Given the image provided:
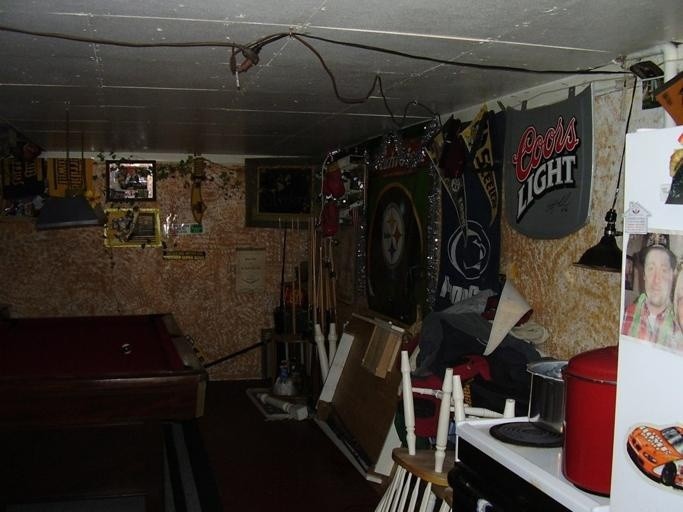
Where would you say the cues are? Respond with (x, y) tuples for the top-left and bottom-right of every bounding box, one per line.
(275, 217), (338, 409)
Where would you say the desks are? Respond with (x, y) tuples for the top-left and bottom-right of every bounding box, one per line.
(1, 312), (209, 511)
(447, 415), (610, 512)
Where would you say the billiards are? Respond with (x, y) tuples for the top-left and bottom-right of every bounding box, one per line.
(120, 343), (131, 354)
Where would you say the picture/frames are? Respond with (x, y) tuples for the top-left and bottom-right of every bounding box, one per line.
(353, 162), (443, 329)
(103, 159), (163, 247)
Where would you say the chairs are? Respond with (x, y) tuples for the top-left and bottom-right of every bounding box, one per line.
(372, 349), (517, 512)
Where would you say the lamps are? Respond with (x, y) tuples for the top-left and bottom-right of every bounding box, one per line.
(36, 99), (101, 233)
(572, 73), (640, 272)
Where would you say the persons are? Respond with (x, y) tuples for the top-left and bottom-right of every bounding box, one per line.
(672, 269), (683, 332)
(620, 243), (682, 346)
